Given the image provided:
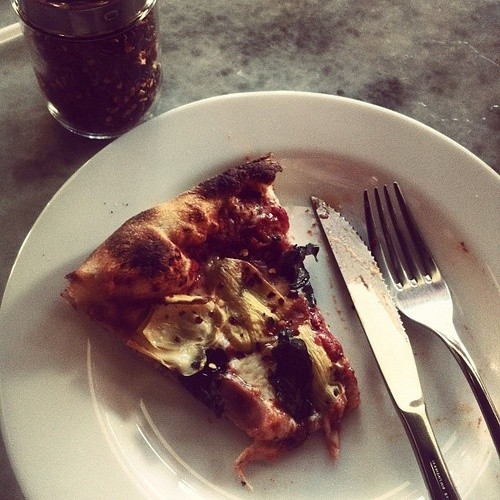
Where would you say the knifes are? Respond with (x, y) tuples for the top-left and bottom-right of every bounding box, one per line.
(308, 193), (465, 500)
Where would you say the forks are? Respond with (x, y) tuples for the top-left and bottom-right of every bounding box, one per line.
(362, 182), (499, 458)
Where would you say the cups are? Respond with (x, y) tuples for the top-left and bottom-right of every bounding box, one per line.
(8, 0), (166, 143)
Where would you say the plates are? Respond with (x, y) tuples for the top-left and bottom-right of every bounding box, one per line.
(1, 86), (500, 499)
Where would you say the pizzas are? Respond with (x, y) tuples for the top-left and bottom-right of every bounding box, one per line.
(60, 153), (361, 494)
